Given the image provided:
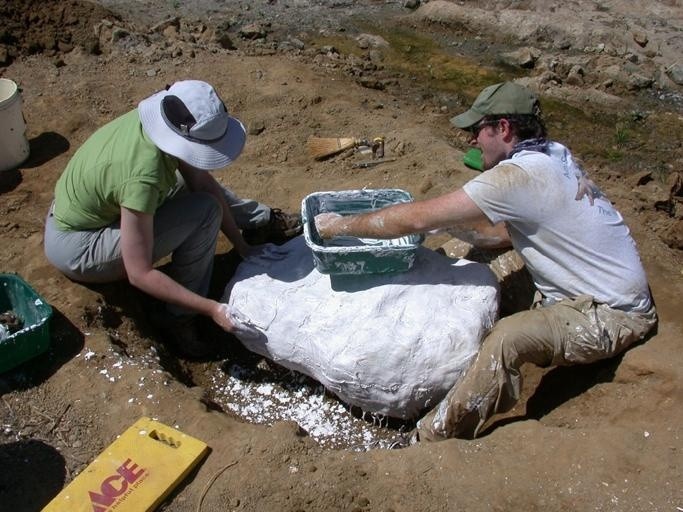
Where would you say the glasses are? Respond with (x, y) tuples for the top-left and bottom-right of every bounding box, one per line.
(469, 119), (500, 138)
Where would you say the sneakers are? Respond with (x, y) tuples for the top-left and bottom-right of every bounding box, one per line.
(163, 315), (211, 364)
(350, 431), (409, 452)
(241, 208), (302, 245)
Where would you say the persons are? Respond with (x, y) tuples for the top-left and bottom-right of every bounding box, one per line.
(43, 78), (303, 359)
(311, 80), (658, 454)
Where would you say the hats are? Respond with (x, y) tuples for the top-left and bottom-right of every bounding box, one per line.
(137, 79), (246, 171)
(448, 79), (540, 132)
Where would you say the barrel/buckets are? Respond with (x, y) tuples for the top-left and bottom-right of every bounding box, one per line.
(0, 77), (31, 173)
